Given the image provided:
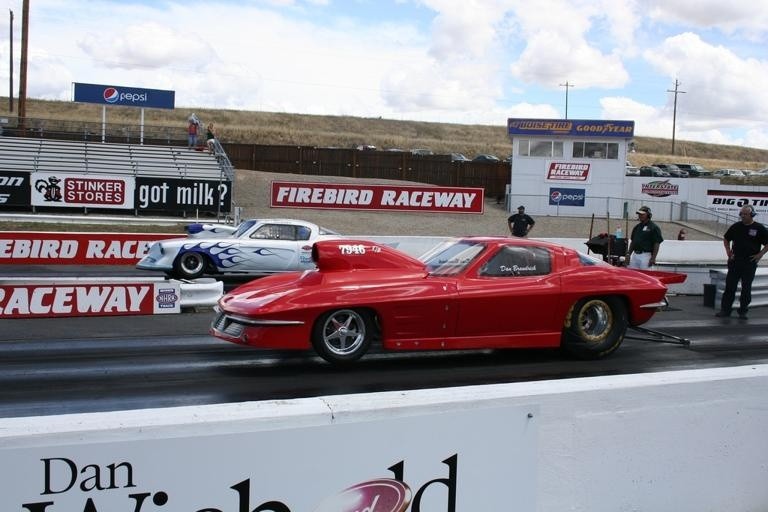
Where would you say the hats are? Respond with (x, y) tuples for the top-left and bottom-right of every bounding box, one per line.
(518, 205), (524, 209)
(635, 205), (651, 215)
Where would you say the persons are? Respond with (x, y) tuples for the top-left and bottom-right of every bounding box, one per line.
(624, 206), (663, 271)
(506, 205), (535, 239)
(715, 204), (768, 320)
(186, 113), (217, 152)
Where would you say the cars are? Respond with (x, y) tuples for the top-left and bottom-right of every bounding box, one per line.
(357, 144), (513, 162)
(626, 161), (768, 178)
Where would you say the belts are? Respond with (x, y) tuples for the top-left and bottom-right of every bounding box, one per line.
(635, 249), (646, 254)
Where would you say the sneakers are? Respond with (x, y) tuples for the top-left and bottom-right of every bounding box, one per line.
(739, 313), (749, 320)
(716, 310), (731, 317)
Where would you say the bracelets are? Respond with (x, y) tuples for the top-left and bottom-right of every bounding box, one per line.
(625, 253), (630, 256)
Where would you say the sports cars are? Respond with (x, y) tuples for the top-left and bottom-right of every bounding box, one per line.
(134, 216), (343, 280)
(208, 237), (691, 364)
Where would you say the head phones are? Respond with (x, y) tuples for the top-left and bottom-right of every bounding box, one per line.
(739, 205), (756, 217)
(647, 208), (652, 219)
